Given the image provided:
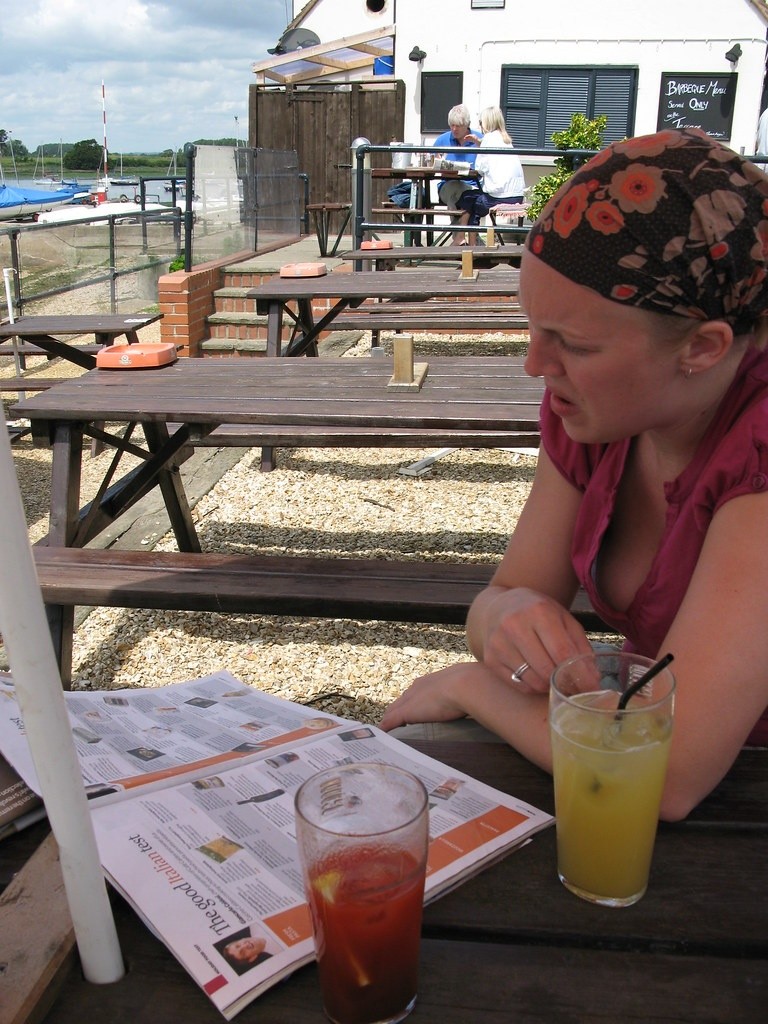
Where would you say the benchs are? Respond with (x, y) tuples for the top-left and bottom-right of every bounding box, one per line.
(2, 303), (545, 447)
(30, 544), (616, 634)
(382, 201), (447, 207)
(306, 203), (380, 257)
(369, 208), (467, 216)
(488, 203), (532, 246)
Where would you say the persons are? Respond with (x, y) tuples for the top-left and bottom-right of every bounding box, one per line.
(755, 107), (768, 172)
(431, 104), (484, 240)
(224, 924), (279, 970)
(377, 128), (768, 821)
(450, 106), (525, 246)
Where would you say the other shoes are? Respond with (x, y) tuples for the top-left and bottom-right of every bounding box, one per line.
(459, 242), (469, 246)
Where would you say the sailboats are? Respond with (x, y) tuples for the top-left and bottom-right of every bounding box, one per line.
(0, 129), (247, 227)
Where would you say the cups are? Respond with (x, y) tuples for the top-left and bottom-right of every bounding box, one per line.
(550, 651), (677, 908)
(294, 762), (431, 1024)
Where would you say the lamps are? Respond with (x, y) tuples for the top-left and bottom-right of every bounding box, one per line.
(725, 43), (742, 63)
(408, 46), (426, 62)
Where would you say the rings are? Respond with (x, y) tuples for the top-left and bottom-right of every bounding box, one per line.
(511, 663), (529, 682)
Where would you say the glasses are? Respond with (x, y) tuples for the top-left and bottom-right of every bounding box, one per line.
(479, 119), (483, 125)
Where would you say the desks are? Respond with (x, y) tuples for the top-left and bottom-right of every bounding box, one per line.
(245, 270), (522, 475)
(8, 354), (546, 692)
(0, 737), (768, 1024)
(0, 312), (165, 457)
(373, 168), (506, 246)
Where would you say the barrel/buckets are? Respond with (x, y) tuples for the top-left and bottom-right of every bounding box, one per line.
(390, 142), (413, 169)
(374, 56), (394, 75)
